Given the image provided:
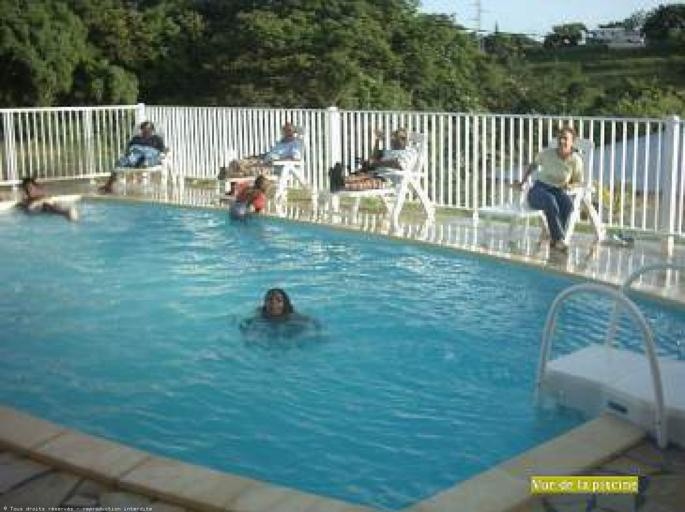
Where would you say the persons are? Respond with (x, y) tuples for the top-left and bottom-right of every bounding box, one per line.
(13, 178), (82, 222)
(217, 120), (303, 182)
(228, 175), (272, 222)
(240, 285), (321, 346)
(327, 129), (418, 197)
(510, 125), (585, 253)
(96, 119), (167, 195)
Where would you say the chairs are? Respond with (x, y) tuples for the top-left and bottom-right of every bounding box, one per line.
(473, 136), (607, 253)
(216, 132), (435, 228)
(113, 124), (176, 198)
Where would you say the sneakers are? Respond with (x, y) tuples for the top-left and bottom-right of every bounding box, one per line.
(326, 162), (346, 193)
(549, 238), (570, 253)
